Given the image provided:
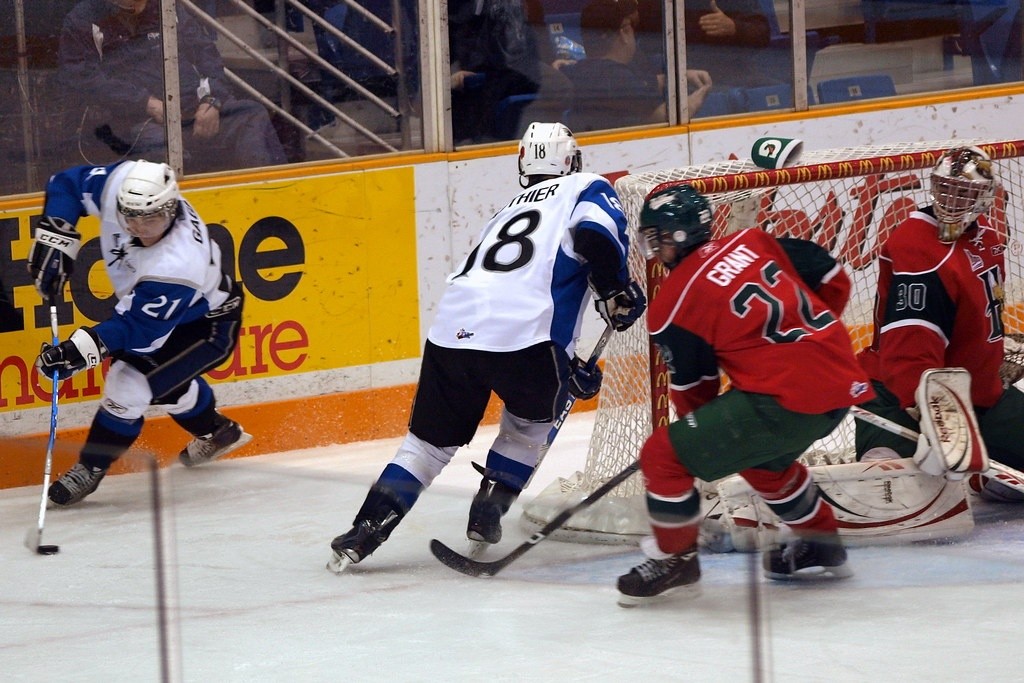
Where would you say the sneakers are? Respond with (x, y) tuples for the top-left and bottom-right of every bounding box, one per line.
(178, 414), (253, 467)
(762, 532), (854, 580)
(325, 484), (405, 576)
(615, 537), (702, 608)
(44, 454), (120, 509)
(965, 473), (1024, 504)
(466, 461), (523, 560)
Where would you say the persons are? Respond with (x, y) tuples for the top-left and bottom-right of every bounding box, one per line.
(29, 160), (251, 504)
(312, 0), (774, 140)
(331, 122), (646, 563)
(852, 145), (1024, 504)
(57, 0), (291, 175)
(618, 185), (878, 598)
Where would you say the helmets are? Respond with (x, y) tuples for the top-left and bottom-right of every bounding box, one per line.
(639, 183), (713, 270)
(116, 159), (180, 237)
(928, 144), (995, 245)
(517, 121), (582, 189)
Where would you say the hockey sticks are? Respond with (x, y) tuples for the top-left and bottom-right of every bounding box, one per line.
(425, 461), (641, 580)
(32, 282), (63, 555)
(849, 402), (1024, 495)
(469, 306), (620, 491)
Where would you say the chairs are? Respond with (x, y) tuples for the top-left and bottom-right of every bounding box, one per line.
(252, 0), (1024, 144)
(35, 67), (61, 164)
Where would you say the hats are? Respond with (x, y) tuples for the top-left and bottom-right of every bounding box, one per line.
(578, 0), (638, 43)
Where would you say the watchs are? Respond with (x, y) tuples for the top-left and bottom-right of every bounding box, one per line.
(199, 95), (223, 110)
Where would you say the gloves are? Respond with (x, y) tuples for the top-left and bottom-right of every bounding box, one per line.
(36, 325), (111, 382)
(27, 215), (82, 301)
(567, 350), (603, 401)
(592, 278), (646, 332)
